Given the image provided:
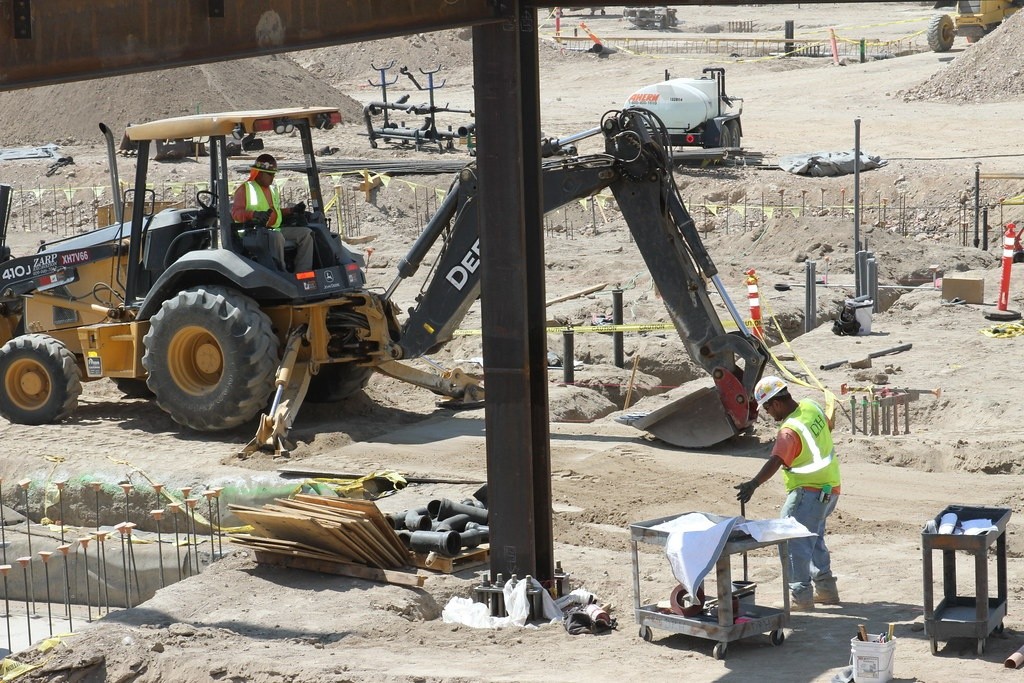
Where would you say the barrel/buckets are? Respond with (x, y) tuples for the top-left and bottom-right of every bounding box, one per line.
(849, 633), (896, 683)
(844, 298), (874, 336)
(717, 596), (739, 624)
(732, 581), (755, 615)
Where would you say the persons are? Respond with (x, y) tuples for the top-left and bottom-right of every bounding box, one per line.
(232, 154), (313, 272)
(734, 376), (841, 611)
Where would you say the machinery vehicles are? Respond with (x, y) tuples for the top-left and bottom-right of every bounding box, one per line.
(928, 0), (1024, 55)
(629, 66), (746, 150)
(0, 104), (772, 453)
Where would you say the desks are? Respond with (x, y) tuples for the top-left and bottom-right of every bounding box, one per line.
(920, 504), (1012, 655)
(628, 510), (790, 659)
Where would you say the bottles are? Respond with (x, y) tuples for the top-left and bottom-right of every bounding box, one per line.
(670, 584), (705, 616)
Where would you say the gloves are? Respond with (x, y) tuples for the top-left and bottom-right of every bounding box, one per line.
(733, 478), (760, 504)
(253, 211), (272, 226)
(292, 202), (305, 216)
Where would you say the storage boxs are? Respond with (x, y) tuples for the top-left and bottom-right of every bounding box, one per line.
(941, 274), (984, 304)
(97, 201), (177, 228)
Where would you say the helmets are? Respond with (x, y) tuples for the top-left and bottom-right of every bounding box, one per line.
(753, 375), (788, 409)
(249, 153), (278, 175)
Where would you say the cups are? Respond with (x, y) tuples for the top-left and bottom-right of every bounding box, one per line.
(384, 481), (489, 556)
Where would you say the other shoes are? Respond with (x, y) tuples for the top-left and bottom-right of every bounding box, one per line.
(812, 589), (840, 604)
(778, 598), (814, 611)
